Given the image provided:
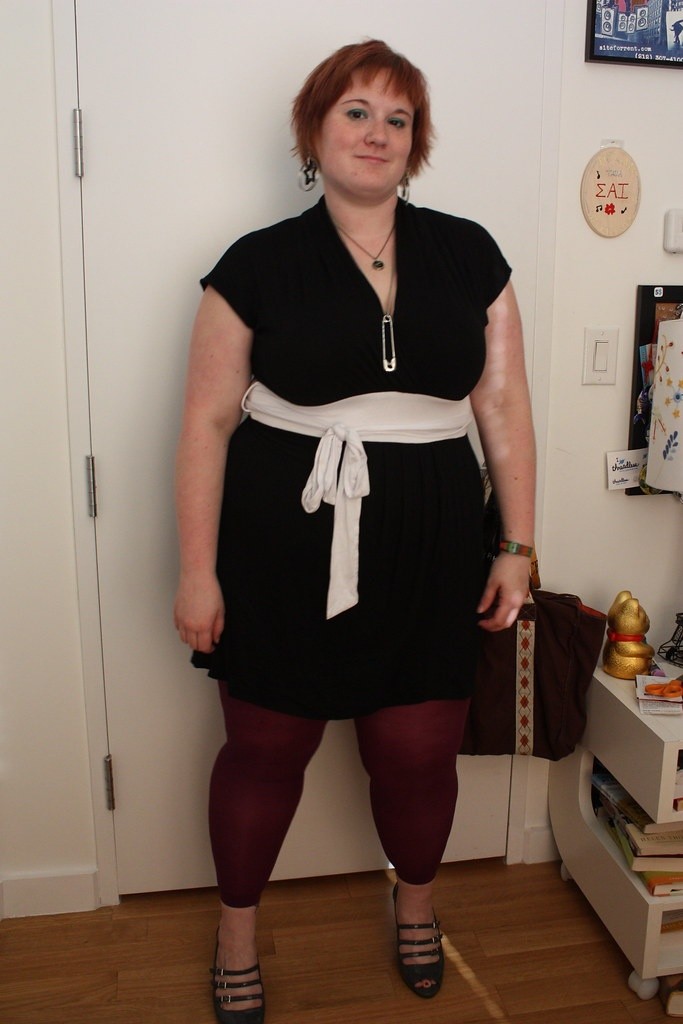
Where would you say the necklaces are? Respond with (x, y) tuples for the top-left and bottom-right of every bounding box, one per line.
(333, 222), (397, 372)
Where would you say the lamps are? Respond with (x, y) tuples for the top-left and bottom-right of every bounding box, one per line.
(644, 319), (683, 668)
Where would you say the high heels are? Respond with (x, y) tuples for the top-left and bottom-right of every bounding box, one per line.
(392, 882), (443, 999)
(209, 926), (266, 1024)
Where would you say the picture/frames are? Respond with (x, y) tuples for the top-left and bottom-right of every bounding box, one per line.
(625, 285), (683, 496)
(584, 0), (683, 69)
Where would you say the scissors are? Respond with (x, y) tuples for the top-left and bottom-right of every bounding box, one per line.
(645, 676), (683, 698)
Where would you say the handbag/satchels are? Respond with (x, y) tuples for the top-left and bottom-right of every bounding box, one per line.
(459, 542), (609, 762)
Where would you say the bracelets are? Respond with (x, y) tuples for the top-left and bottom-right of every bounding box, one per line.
(500, 540), (533, 558)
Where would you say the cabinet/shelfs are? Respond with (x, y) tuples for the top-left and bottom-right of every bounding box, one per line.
(546, 667), (683, 980)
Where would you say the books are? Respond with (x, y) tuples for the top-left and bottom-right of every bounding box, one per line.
(592, 770), (683, 897)
(659, 973), (683, 1017)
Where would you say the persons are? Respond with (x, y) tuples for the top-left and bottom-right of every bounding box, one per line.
(174, 40), (537, 1024)
